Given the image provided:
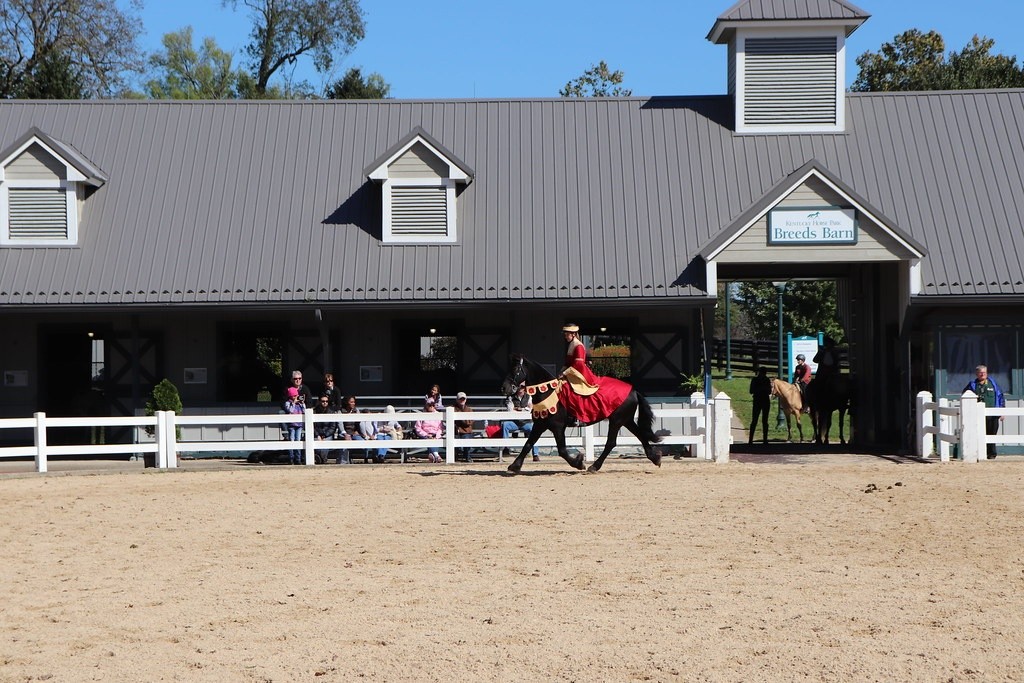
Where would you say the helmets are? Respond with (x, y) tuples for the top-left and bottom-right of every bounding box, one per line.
(796, 354), (806, 361)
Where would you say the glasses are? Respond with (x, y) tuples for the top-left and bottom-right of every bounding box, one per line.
(429, 405), (434, 407)
(322, 401), (328, 403)
(293, 378), (301, 380)
(458, 398), (465, 401)
(327, 380), (333, 383)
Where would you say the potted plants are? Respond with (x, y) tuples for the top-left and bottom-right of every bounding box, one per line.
(143, 379), (183, 467)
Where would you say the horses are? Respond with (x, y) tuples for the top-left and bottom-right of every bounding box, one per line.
(500, 350), (664, 475)
(769, 344), (846, 447)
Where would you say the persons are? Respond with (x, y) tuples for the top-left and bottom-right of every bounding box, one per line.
(557, 323), (599, 396)
(319, 374), (341, 411)
(414, 384), (446, 463)
(452, 391), (474, 463)
(279, 370), (313, 440)
(313, 393), (338, 464)
(813, 336), (842, 379)
(961, 365), (1005, 460)
(500, 381), (541, 462)
(337, 395), (370, 465)
(792, 355), (812, 394)
(284, 388), (306, 465)
(359, 404), (403, 464)
(747, 366), (772, 445)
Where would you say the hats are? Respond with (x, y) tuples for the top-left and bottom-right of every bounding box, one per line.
(288, 387), (298, 397)
(457, 392), (467, 400)
(426, 398), (434, 403)
(563, 323), (579, 331)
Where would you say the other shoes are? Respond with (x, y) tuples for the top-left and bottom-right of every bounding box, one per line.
(372, 455), (384, 463)
(533, 455), (539, 461)
(428, 454), (441, 463)
(289, 459), (294, 465)
(498, 447), (510, 457)
(336, 459), (347, 464)
(296, 461), (302, 465)
